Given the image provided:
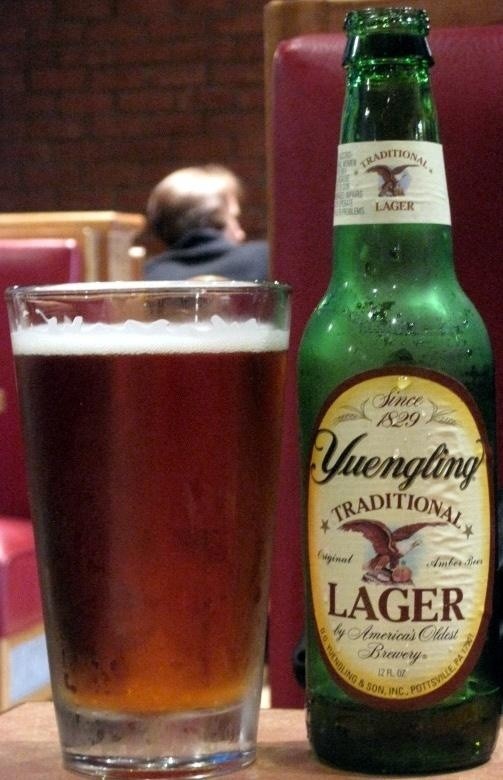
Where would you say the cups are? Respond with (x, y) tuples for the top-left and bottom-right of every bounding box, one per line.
(7, 281), (293, 777)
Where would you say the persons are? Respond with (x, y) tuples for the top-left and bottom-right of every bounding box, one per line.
(140, 167), (269, 321)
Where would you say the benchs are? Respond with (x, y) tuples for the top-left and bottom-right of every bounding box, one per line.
(257, 2), (503, 712)
(0, 211), (143, 712)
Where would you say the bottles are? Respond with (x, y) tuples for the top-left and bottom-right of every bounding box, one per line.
(294, 4), (493, 777)
